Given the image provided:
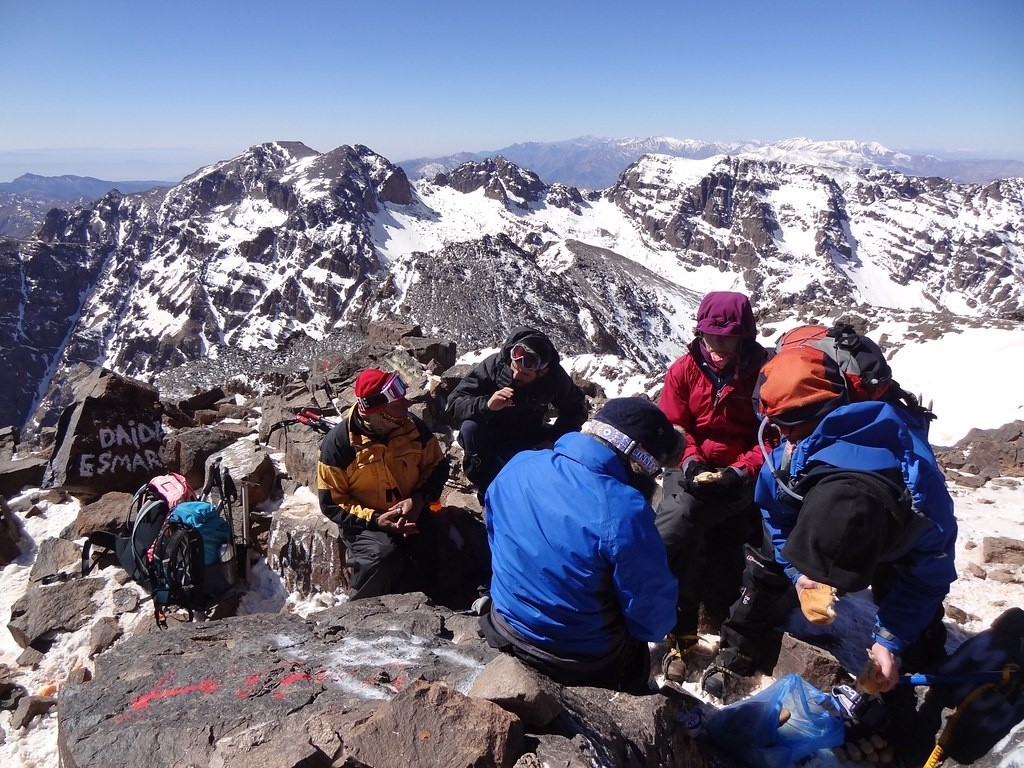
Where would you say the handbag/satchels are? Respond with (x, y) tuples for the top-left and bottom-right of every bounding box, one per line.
(706, 674), (846, 768)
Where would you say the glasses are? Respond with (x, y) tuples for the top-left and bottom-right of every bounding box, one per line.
(357, 369), (409, 410)
(509, 343), (550, 370)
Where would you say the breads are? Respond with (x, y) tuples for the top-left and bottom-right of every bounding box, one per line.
(856, 648), (888, 693)
(799, 581), (839, 624)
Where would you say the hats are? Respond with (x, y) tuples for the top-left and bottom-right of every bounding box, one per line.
(695, 291), (757, 338)
(513, 336), (554, 373)
(594, 397), (677, 472)
(353, 368), (396, 415)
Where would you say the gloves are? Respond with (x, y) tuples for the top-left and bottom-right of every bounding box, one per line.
(678, 461), (742, 503)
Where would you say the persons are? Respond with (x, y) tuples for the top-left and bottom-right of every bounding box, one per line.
(478, 397), (679, 684)
(317, 368), (471, 603)
(447, 328), (589, 492)
(654, 291), (960, 709)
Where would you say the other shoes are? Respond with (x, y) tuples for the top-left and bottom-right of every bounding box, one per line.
(661, 637), (694, 682)
(700, 658), (739, 699)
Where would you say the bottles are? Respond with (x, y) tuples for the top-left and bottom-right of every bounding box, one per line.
(219, 544), (236, 586)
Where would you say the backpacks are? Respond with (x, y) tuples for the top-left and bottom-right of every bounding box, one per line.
(150, 500), (237, 612)
(117, 472), (198, 586)
(751, 321), (902, 502)
